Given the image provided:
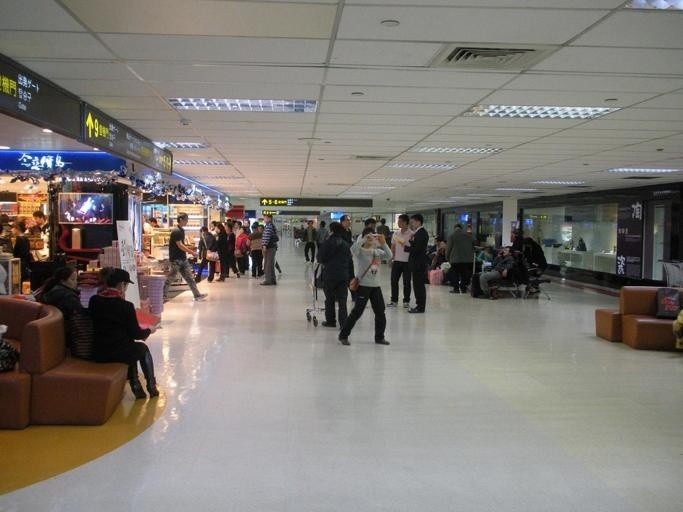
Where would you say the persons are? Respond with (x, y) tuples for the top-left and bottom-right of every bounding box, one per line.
(445, 223), (474, 294)
(147, 213), (283, 282)
(0, 209), (52, 297)
(316, 221), (353, 328)
(336, 227), (393, 346)
(161, 213), (208, 303)
(59, 195), (111, 222)
(293, 214), (587, 314)
(258, 213), (278, 286)
(40, 264), (160, 399)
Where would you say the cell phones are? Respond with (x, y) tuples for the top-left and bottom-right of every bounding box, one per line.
(370, 233), (379, 240)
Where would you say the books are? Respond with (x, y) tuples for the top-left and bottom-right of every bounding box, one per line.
(135, 307), (160, 342)
(392, 234), (405, 245)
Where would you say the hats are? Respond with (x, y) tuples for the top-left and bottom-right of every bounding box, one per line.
(111, 268), (134, 284)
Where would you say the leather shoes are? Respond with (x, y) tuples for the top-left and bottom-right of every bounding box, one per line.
(375, 339), (389, 344)
(450, 289), (466, 293)
(322, 321), (350, 345)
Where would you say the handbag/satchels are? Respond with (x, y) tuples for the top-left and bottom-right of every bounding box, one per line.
(349, 278), (358, 291)
(655, 288), (680, 320)
(428, 263), (444, 284)
(0, 340), (18, 372)
(206, 249), (220, 261)
(314, 264), (325, 289)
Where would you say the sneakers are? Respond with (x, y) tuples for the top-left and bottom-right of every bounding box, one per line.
(387, 301), (424, 313)
(194, 294), (207, 299)
(240, 271), (276, 285)
(195, 271), (240, 285)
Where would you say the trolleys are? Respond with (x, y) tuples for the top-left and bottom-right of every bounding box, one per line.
(304, 260), (326, 328)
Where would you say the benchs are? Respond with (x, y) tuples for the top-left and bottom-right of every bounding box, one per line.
(0, 294), (131, 430)
(595, 286), (683, 351)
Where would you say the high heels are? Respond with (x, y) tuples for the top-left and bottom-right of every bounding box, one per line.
(128, 376), (145, 398)
(146, 378), (159, 398)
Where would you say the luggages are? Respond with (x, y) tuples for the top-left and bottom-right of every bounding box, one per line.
(471, 251), (484, 297)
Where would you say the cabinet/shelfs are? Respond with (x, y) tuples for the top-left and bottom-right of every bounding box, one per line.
(0, 193), (49, 296)
(137, 202), (223, 292)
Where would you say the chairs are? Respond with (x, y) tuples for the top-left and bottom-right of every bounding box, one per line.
(489, 249), (551, 300)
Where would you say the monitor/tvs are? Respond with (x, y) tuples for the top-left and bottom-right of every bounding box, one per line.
(55, 191), (115, 228)
